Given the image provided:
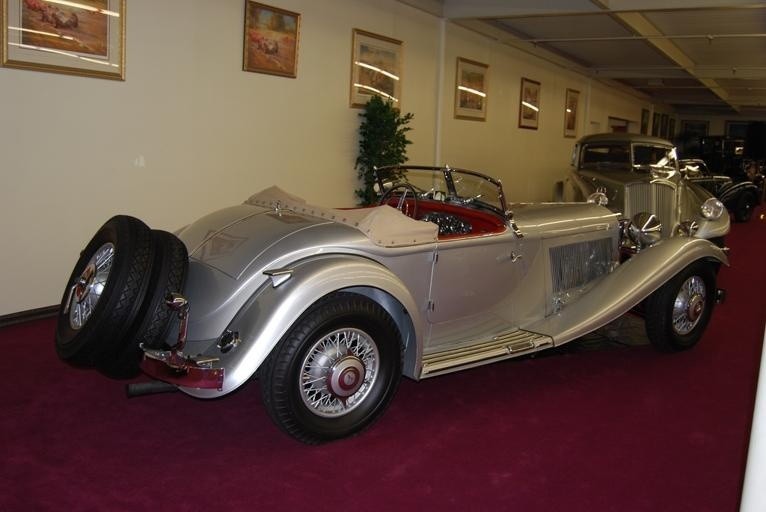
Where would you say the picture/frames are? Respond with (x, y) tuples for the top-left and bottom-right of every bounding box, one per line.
(350, 28), (404, 112)
(638, 108), (676, 140)
(244, 2), (299, 80)
(0, 1), (126, 82)
(453, 55), (581, 137)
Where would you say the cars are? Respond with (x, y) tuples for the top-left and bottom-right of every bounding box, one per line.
(127, 163), (730, 445)
(562, 133), (759, 256)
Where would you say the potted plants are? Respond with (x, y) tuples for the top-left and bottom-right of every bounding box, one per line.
(354, 93), (415, 206)
